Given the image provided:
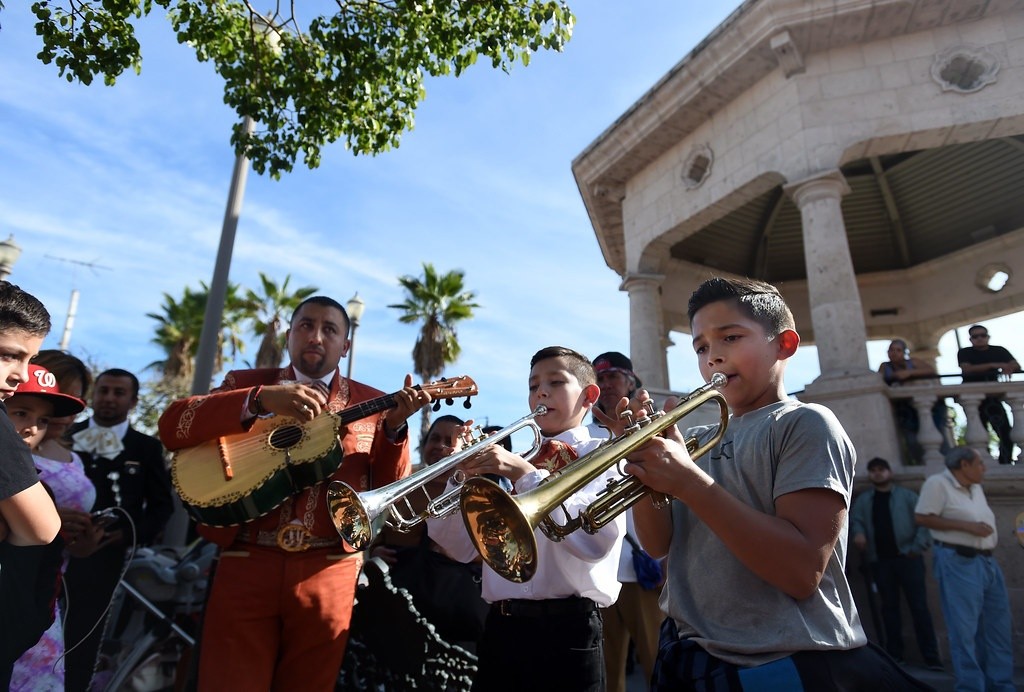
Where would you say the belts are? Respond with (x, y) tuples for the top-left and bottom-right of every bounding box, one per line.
(491, 597), (598, 617)
(933, 540), (993, 558)
(239, 523), (340, 552)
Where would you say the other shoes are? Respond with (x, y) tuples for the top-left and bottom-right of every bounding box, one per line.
(922, 656), (945, 671)
(890, 655), (908, 665)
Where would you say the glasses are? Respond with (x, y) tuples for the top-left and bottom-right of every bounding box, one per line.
(970, 333), (988, 339)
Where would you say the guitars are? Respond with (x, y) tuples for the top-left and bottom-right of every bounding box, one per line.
(170, 375), (480, 530)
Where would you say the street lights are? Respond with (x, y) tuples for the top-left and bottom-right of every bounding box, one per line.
(192, 10), (285, 395)
(1, 233), (23, 280)
(346, 291), (364, 379)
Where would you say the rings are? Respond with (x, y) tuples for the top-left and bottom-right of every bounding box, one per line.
(301, 405), (307, 412)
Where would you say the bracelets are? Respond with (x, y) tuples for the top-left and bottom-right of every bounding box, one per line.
(253, 384), (266, 415)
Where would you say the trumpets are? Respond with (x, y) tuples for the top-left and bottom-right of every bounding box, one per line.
(457, 371), (732, 585)
(327, 403), (549, 552)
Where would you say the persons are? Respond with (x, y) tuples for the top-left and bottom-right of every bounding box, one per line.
(590, 276), (940, 691)
(878, 340), (934, 466)
(957, 324), (1021, 464)
(158, 295), (432, 691)
(914, 445), (1016, 692)
(370, 346), (626, 692)
(851, 457), (944, 671)
(0, 277), (109, 691)
(62, 368), (175, 691)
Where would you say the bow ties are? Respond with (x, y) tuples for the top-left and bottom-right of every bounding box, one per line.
(511, 439), (579, 495)
(72, 426), (125, 454)
(302, 380), (330, 403)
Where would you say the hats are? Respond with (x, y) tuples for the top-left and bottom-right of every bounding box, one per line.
(592, 352), (642, 394)
(14, 365), (85, 418)
